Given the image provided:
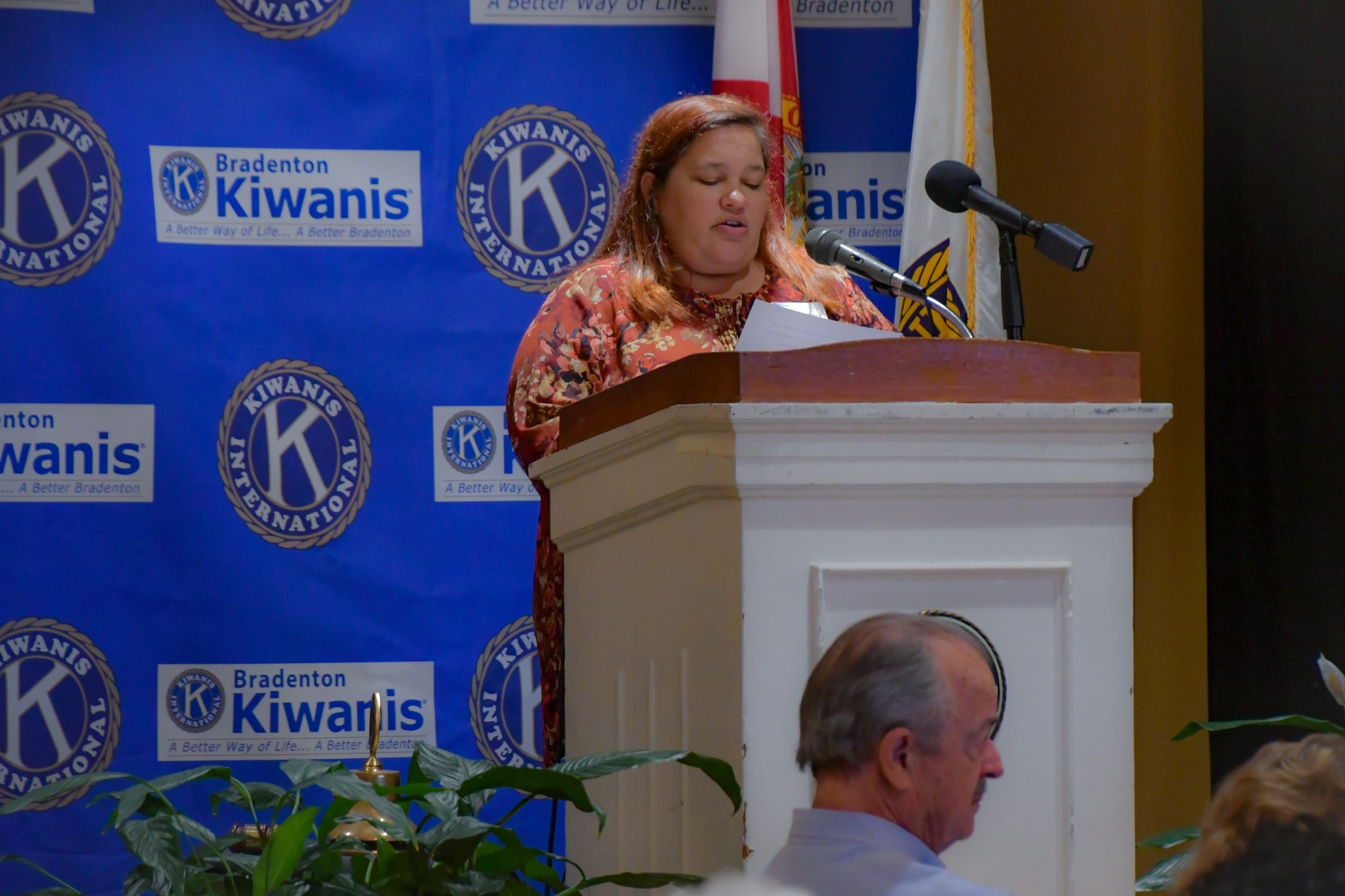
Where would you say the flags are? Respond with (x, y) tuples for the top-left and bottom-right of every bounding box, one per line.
(893, 0), (1027, 346)
(709, 0), (810, 253)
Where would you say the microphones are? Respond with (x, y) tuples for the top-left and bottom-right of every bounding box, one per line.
(805, 226), (927, 300)
(925, 160), (1094, 272)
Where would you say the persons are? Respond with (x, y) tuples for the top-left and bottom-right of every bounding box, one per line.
(759, 614), (1004, 896)
(1145, 732), (1345, 896)
(495, 92), (905, 771)
